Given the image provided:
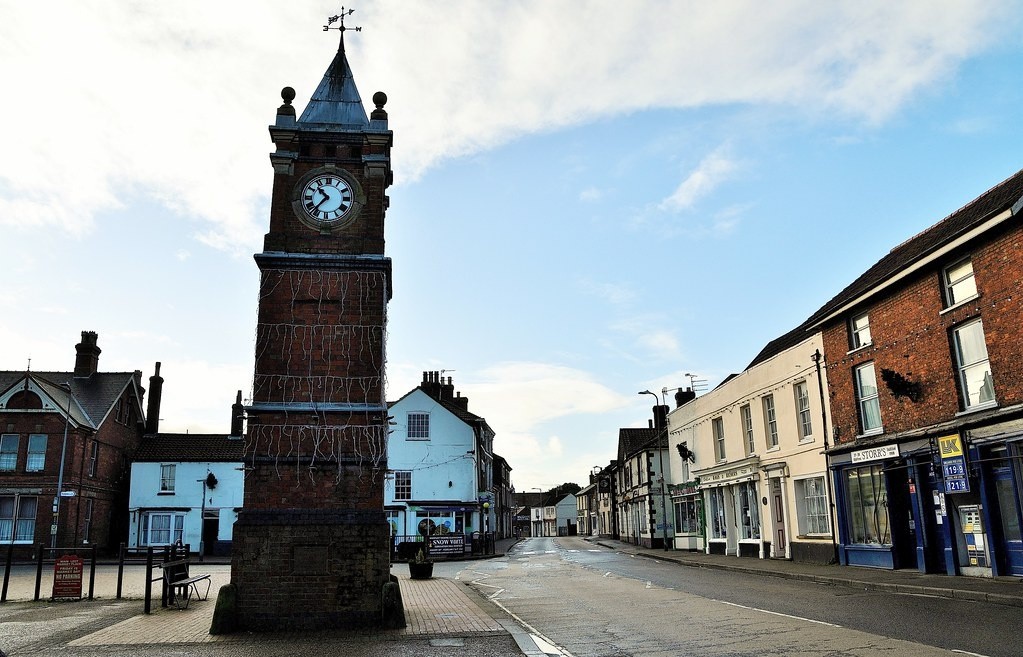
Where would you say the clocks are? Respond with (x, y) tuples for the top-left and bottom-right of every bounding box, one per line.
(299, 170), (355, 225)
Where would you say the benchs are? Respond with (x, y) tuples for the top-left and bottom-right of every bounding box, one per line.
(159, 557), (212, 610)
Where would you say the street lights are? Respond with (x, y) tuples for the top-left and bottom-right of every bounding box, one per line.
(48, 381), (73, 565)
(532, 487), (543, 537)
(638, 390), (669, 552)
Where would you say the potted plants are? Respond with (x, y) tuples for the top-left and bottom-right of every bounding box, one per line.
(409, 549), (434, 580)
